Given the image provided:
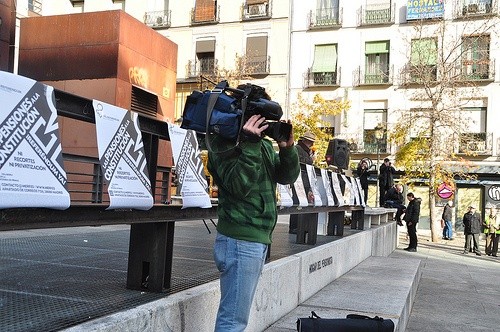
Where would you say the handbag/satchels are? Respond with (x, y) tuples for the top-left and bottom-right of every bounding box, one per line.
(441, 218), (444, 228)
(297, 311), (394, 332)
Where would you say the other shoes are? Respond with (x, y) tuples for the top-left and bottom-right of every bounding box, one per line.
(463, 252), (468, 254)
(289, 229), (306, 235)
(395, 217), (403, 226)
(403, 247), (416, 252)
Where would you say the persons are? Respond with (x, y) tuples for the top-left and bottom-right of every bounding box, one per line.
(383, 183), (406, 226)
(356, 161), (370, 204)
(463, 205), (482, 256)
(483, 207), (500, 256)
(442, 200), (454, 240)
(379, 158), (395, 208)
(207, 83), (301, 332)
(402, 193), (422, 252)
(289, 132), (316, 234)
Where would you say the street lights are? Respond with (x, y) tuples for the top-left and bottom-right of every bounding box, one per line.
(373, 123), (385, 207)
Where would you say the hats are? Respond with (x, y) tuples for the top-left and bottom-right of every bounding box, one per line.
(299, 132), (315, 141)
(471, 205), (476, 208)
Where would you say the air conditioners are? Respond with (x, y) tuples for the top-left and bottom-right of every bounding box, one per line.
(145, 10), (168, 27)
(248, 4), (266, 17)
(467, 3), (486, 15)
(467, 142), (485, 151)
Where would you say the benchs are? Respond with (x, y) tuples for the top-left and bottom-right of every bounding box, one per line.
(364, 207), (397, 227)
